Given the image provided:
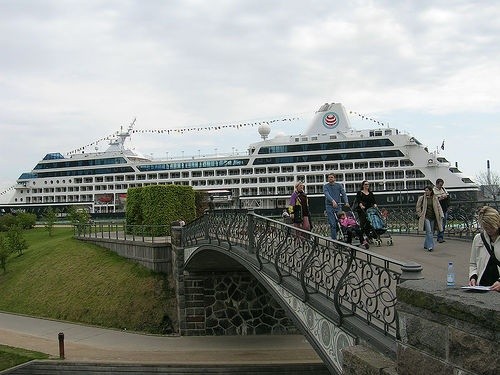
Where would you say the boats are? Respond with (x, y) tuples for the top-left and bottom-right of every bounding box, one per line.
(96, 198), (112, 203)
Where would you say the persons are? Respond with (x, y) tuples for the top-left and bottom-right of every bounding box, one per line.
(468, 206), (500, 292)
(323, 173), (387, 249)
(417, 178), (450, 251)
(289, 182), (314, 240)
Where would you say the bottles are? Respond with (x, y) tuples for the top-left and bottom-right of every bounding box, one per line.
(446, 263), (455, 286)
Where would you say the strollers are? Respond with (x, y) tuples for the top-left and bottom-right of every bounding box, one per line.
(332, 203), (370, 250)
(361, 206), (393, 247)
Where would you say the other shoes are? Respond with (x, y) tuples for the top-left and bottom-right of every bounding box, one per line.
(428, 247), (432, 251)
(437, 239), (445, 243)
(424, 247), (427, 249)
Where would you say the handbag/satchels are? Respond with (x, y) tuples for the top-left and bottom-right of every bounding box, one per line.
(352, 197), (359, 212)
(289, 199), (303, 223)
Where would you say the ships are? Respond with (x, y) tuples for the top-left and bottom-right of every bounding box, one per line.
(0, 102), (482, 220)
